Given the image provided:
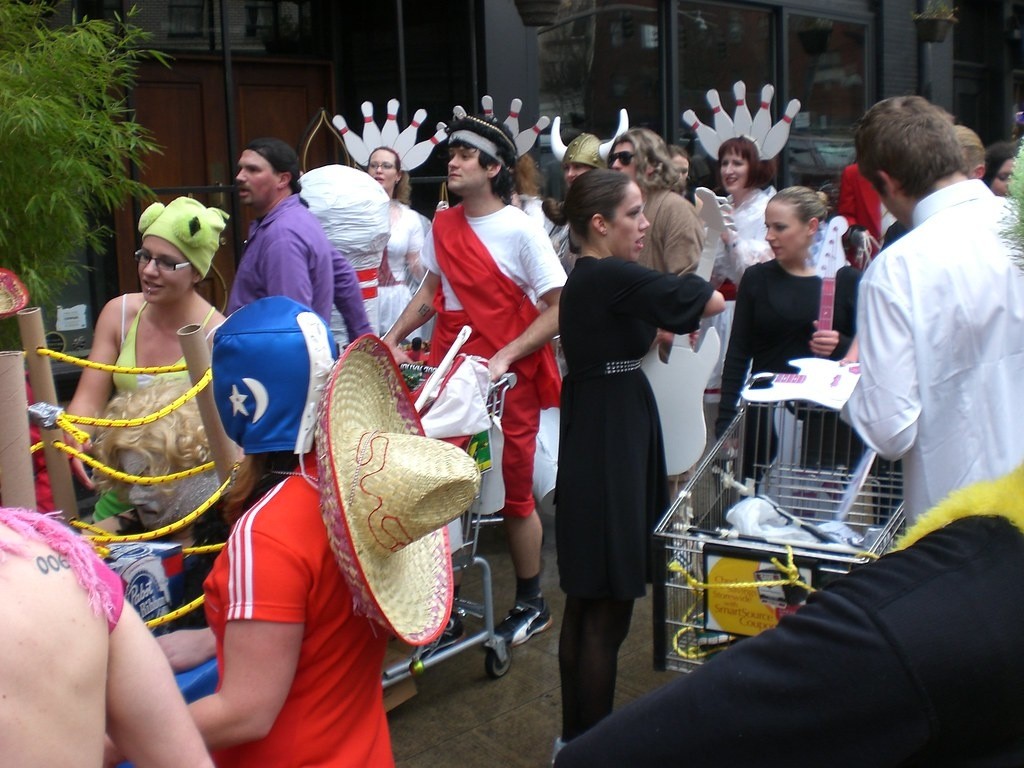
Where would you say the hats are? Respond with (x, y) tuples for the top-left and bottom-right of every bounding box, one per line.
(562, 132), (604, 168)
(607, 150), (635, 169)
(138, 196), (230, 281)
(211, 294), (337, 454)
(316, 331), (480, 645)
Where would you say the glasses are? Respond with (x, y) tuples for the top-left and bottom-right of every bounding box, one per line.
(135, 248), (190, 272)
(368, 161), (398, 170)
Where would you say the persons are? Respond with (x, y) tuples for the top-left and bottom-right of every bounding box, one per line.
(0, 94), (1022, 768)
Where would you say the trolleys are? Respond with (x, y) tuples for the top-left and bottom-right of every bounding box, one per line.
(652, 356), (907, 673)
(379, 361), (518, 689)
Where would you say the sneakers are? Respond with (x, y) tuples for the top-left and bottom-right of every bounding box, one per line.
(494, 592), (553, 648)
